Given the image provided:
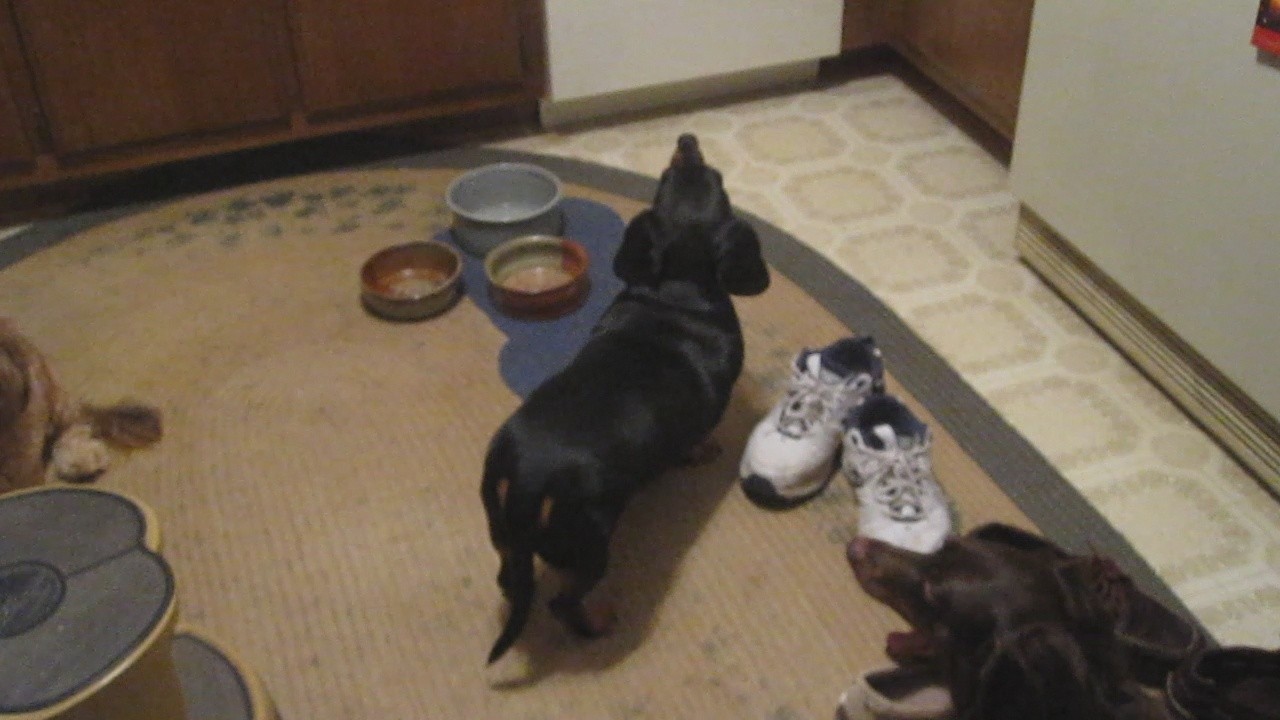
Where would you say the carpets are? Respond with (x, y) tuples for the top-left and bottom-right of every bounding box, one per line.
(1, 142), (1223, 720)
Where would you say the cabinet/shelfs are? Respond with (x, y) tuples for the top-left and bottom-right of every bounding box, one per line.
(0, 0), (549, 183)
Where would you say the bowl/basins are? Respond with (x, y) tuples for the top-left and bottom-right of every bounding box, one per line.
(445, 164), (564, 255)
(485, 236), (588, 310)
(363, 239), (459, 318)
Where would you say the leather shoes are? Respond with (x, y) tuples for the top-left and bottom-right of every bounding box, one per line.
(967, 522), (1208, 689)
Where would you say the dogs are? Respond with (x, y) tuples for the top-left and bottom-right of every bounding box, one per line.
(482, 132), (771, 667)
(845, 523), (1280, 720)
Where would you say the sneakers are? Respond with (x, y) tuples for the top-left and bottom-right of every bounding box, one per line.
(838, 663), (953, 719)
(736, 336), (887, 508)
(843, 384), (955, 556)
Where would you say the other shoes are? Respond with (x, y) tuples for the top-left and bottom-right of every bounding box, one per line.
(1166, 645), (1280, 720)
